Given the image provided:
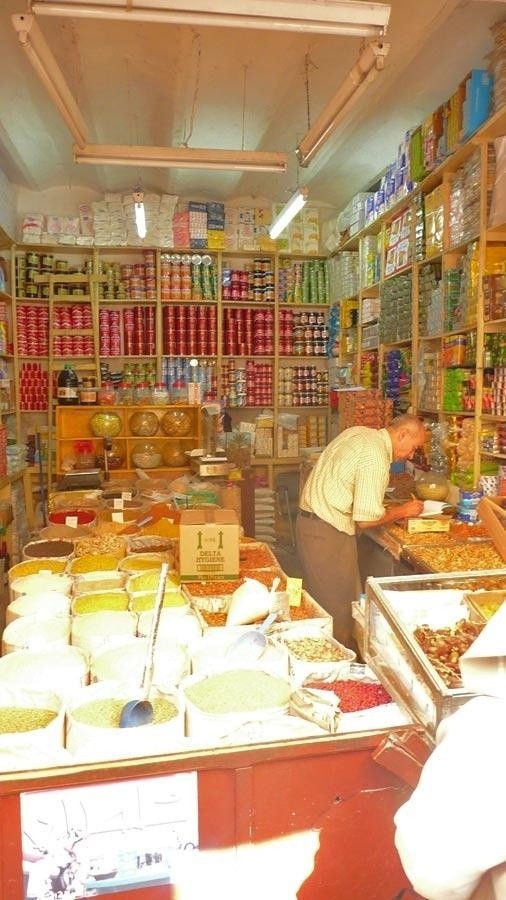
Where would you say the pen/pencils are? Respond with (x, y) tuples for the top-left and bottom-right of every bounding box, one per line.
(410, 492), (417, 500)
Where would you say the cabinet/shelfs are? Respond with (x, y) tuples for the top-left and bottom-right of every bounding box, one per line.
(332, 103), (506, 512)
(15, 246), (332, 530)
(0, 226), (16, 574)
(53, 405), (205, 469)
(0, 534), (424, 900)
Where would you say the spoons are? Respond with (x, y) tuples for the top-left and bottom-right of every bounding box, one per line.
(120, 563), (169, 728)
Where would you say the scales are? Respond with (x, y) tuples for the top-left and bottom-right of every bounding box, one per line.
(190, 457), (230, 480)
(55, 468), (104, 490)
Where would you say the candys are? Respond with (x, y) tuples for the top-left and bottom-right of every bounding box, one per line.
(98, 393), (116, 405)
(89, 414), (122, 436)
(153, 391), (169, 406)
(73, 450), (95, 468)
(170, 393), (189, 405)
(164, 449), (190, 466)
(131, 453), (161, 468)
(135, 394), (152, 405)
(131, 414), (157, 436)
(96, 455), (123, 470)
(162, 413), (191, 435)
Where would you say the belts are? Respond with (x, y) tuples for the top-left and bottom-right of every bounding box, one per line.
(298, 508), (321, 520)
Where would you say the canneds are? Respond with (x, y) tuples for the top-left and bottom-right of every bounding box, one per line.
(111, 346), (121, 356)
(39, 343), (49, 356)
(279, 367), (285, 381)
(38, 307), (48, 318)
(285, 381), (292, 394)
(17, 306), (26, 317)
(26, 307), (37, 317)
(0, 302), (13, 356)
(17, 317), (27, 330)
(16, 330), (27, 342)
(110, 337), (120, 347)
(100, 310), (109, 325)
(101, 346), (110, 355)
(28, 342), (38, 356)
(38, 319), (48, 330)
(279, 393), (285, 407)
(111, 312), (118, 326)
(28, 330), (37, 342)
(278, 381), (284, 393)
(38, 331), (48, 342)
(15, 255), (26, 300)
(53, 336), (93, 357)
(17, 341), (28, 355)
(53, 307), (92, 328)
(100, 326), (109, 337)
(111, 326), (118, 337)
(100, 338), (109, 348)
(285, 394), (293, 407)
(285, 367), (292, 382)
(27, 317), (38, 331)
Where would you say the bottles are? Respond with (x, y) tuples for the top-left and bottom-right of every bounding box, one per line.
(227, 343), (236, 356)
(198, 319), (207, 330)
(146, 343), (156, 356)
(28, 394), (33, 402)
(29, 378), (34, 386)
(84, 260), (95, 279)
(198, 342), (208, 355)
(313, 341), (322, 356)
(295, 326), (304, 341)
(26, 285), (37, 299)
(222, 286), (231, 301)
(117, 291), (125, 298)
(121, 264), (132, 279)
(56, 260), (67, 272)
(229, 371), (235, 382)
(33, 371), (39, 378)
(321, 326), (329, 341)
(165, 330), (176, 342)
(308, 311), (316, 326)
(28, 363), (34, 370)
(324, 383), (329, 392)
(245, 309), (252, 320)
(208, 320), (216, 330)
(187, 342), (197, 355)
(236, 332), (245, 344)
(41, 284), (49, 297)
(80, 376), (98, 406)
(231, 285), (239, 301)
(222, 269), (231, 286)
(262, 289), (266, 303)
(182, 277), (191, 287)
(197, 330), (208, 342)
(145, 264), (155, 277)
(310, 365), (316, 379)
(124, 363), (134, 376)
(203, 390), (217, 403)
(165, 306), (175, 317)
(299, 393), (305, 407)
(297, 379), (304, 393)
(134, 263), (144, 279)
(171, 266), (180, 277)
(265, 272), (274, 288)
(253, 258), (262, 273)
(247, 360), (274, 407)
(290, 367), (297, 379)
(20, 402), (25, 410)
(280, 257), (328, 306)
(187, 318), (196, 330)
(34, 363), (40, 370)
(34, 395), (40, 402)
(316, 312), (325, 326)
(166, 342), (176, 355)
(130, 276), (141, 289)
(165, 317), (174, 331)
(177, 342), (186, 355)
(99, 261), (125, 291)
(221, 363), (229, 402)
(292, 380), (298, 393)
(39, 371), (46, 378)
(254, 308), (266, 356)
(43, 402), (47, 410)
(236, 309), (243, 320)
(124, 279), (129, 291)
(105, 291), (114, 300)
(34, 378), (41, 386)
(229, 396), (237, 407)
(312, 393), (317, 406)
(135, 306), (144, 318)
(304, 366), (310, 379)
(58, 364), (79, 405)
(293, 393), (299, 407)
(162, 358), (218, 389)
(285, 324), (293, 339)
(101, 362), (122, 391)
(316, 371), (323, 380)
(245, 320), (252, 332)
(297, 367), (304, 379)
(321, 341), (329, 357)
(240, 285), (246, 300)
(187, 306), (196, 318)
(171, 276), (181, 289)
(294, 312), (300, 326)
(261, 274), (266, 287)
(237, 344), (247, 355)
(145, 306), (155, 318)
(237, 393), (247, 407)
(186, 331), (196, 342)
(247, 271), (253, 285)
(26, 386), (34, 395)
(208, 306), (216, 319)
(20, 371), (25, 378)
(227, 319), (235, 331)
(285, 310), (292, 324)
(38, 402), (43, 410)
(176, 306), (185, 318)
(40, 378), (47, 387)
(239, 271), (247, 285)
(231, 271), (239, 285)
(301, 311), (309, 326)
(135, 363), (146, 376)
(27, 269), (40, 282)
(311, 380), (317, 392)
(21, 386), (26, 395)
(135, 375), (146, 388)
(124, 308), (135, 356)
(54, 283), (68, 296)
(322, 369), (331, 383)
(208, 342), (217, 355)
(40, 254), (53, 269)
(146, 277), (156, 290)
(280, 326), (285, 339)
(278, 339), (285, 356)
(145, 251), (154, 264)
(23, 395), (28, 403)
(229, 359), (235, 371)
(285, 339), (295, 355)
(146, 331), (155, 344)
(182, 289), (191, 300)
(262, 257), (271, 272)
(294, 341), (305, 356)
(254, 289), (262, 302)
(98, 284), (104, 297)
(230, 383), (236, 393)
(253, 273), (262, 289)
(208, 331), (217, 342)
(266, 340), (275, 356)
(180, 266), (190, 277)
(304, 326), (313, 341)
(117, 393), (134, 406)
(318, 395), (323, 406)
(23, 378), (29, 386)
(171, 288), (180, 300)
(25, 250), (40, 268)
(237, 368), (247, 381)
(324, 394), (330, 406)
(266, 287), (275, 303)
(279, 309), (284, 325)
(130, 288), (141, 300)
(22, 363), (28, 370)
(318, 382), (324, 393)
(41, 395), (47, 402)
(197, 306), (206, 319)
(72, 285), (84, 296)
(226, 309), (234, 319)
(236, 320), (244, 332)
(178, 331), (186, 342)
(305, 393), (311, 406)
(135, 331), (144, 343)
(305, 379), (311, 393)
(33, 386), (40, 394)
(227, 332), (235, 343)
(147, 318), (155, 330)
(25, 371), (33, 378)
(304, 341), (314, 357)
(160, 289), (170, 300)
(245, 332), (253, 344)
(247, 344), (253, 356)
(136, 319), (144, 331)
(161, 276), (169, 289)
(136, 343), (145, 356)
(175, 318), (186, 329)
(146, 375), (157, 387)
(248, 287), (254, 302)
(313, 325), (321, 342)
(25, 402), (31, 410)
(146, 363), (156, 374)
(161, 264), (171, 277)
(266, 309), (274, 324)
(147, 289), (157, 299)
(124, 375), (134, 387)
(265, 325), (274, 340)
(40, 387), (46, 395)
(33, 402), (38, 410)
(192, 264), (218, 301)
(237, 380), (247, 393)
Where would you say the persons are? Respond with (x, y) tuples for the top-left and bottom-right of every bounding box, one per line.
(295, 415), (425, 662)
